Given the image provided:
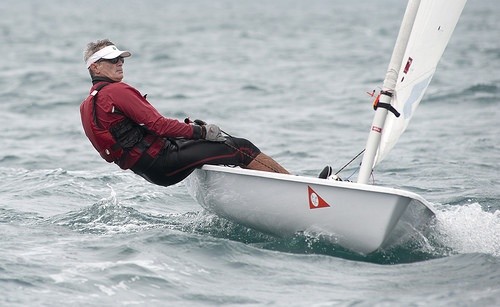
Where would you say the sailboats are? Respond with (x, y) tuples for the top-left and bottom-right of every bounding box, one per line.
(182, 1), (468, 261)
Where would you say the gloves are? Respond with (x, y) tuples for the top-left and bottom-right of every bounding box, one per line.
(204, 125), (220, 140)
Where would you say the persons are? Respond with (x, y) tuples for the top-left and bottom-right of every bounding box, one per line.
(80, 41), (292, 186)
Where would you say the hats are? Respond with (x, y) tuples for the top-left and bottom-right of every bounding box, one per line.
(86, 45), (131, 69)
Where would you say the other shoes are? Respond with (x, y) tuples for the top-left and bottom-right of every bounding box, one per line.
(318, 166), (332, 179)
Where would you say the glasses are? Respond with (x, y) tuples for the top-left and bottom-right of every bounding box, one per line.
(94, 57), (125, 63)
